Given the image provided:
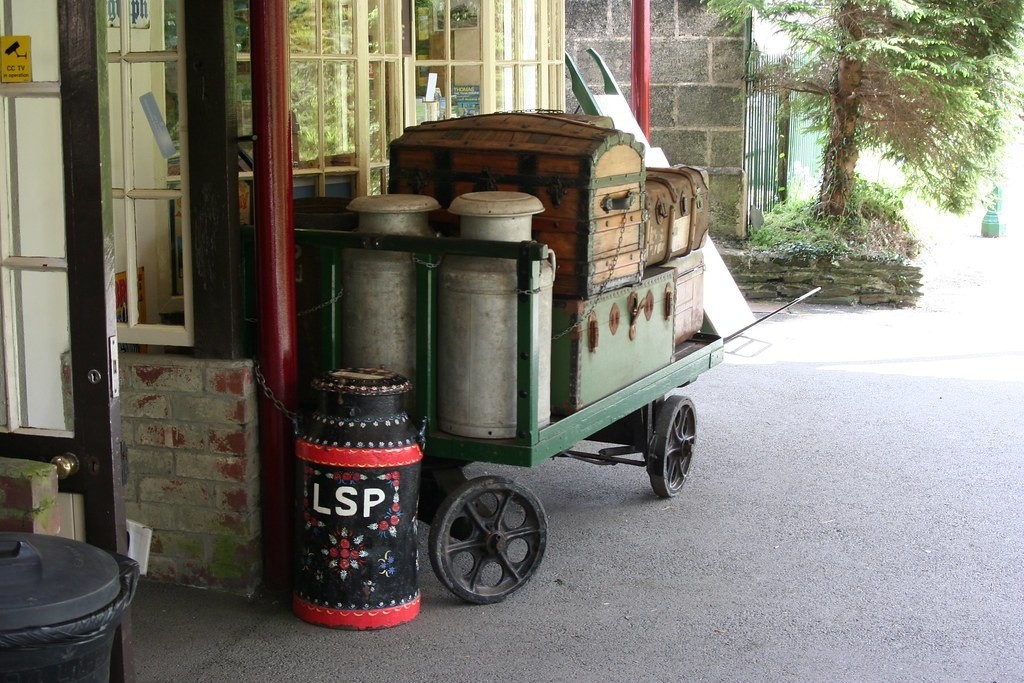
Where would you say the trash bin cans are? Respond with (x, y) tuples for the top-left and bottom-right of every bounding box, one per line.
(0, 531), (140, 683)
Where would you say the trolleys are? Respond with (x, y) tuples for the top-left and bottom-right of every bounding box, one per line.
(298, 229), (823, 607)
